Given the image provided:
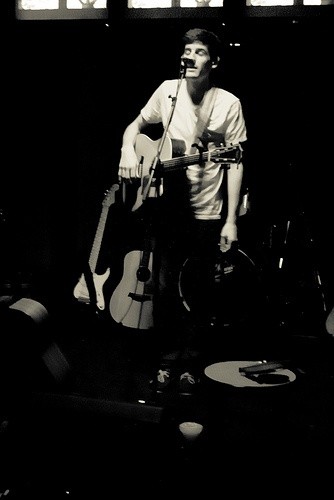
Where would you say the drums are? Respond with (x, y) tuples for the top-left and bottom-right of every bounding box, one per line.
(201, 359), (304, 428)
(178, 244), (257, 329)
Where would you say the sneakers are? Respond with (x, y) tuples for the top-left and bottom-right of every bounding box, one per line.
(178, 371), (198, 396)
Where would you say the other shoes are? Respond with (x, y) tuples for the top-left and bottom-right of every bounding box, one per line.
(151, 368), (174, 394)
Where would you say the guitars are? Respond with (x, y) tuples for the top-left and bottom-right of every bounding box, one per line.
(121, 131), (243, 213)
(110, 231), (154, 331)
(73, 182), (119, 312)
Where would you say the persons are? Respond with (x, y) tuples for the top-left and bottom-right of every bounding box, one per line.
(116, 26), (247, 409)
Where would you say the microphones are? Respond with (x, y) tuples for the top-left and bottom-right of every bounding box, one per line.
(182, 56), (195, 68)
